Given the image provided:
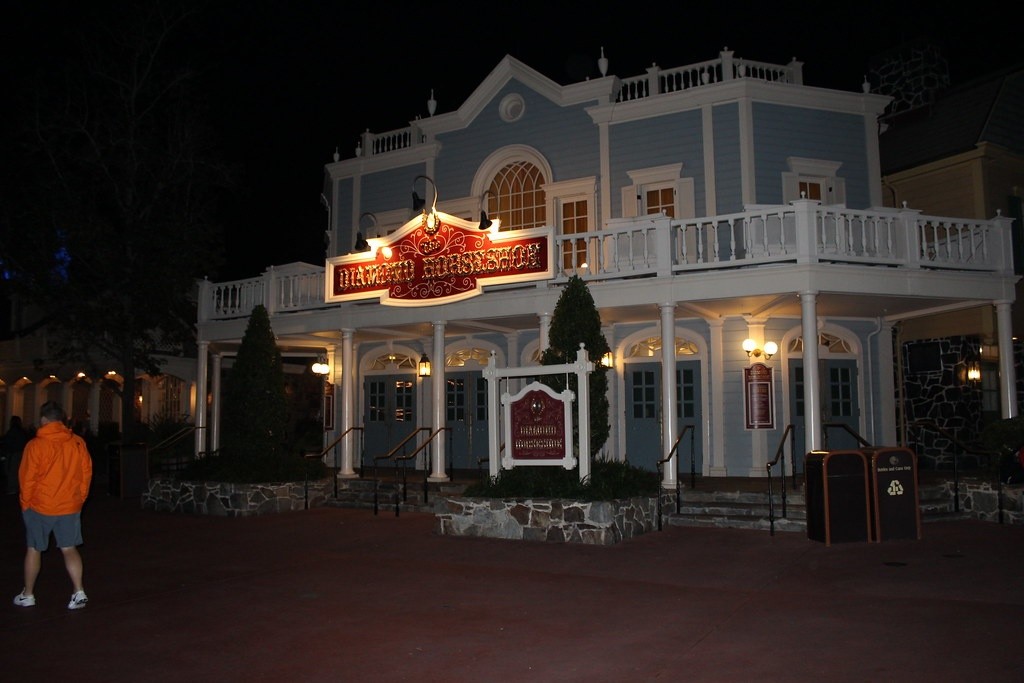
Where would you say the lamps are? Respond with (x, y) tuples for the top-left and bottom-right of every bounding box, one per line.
(411, 175), (440, 233)
(743, 338), (777, 361)
(601, 347), (613, 368)
(478, 191), (503, 233)
(418, 354), (432, 377)
(311, 352), (329, 374)
(355, 212), (380, 251)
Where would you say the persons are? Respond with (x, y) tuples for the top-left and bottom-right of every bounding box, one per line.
(13, 401), (93, 610)
(5, 416), (28, 496)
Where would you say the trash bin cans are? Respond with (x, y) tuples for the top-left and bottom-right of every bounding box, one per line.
(105, 442), (150, 499)
(804, 449), (874, 545)
(860, 446), (923, 543)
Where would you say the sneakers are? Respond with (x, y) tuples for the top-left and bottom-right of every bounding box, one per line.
(68, 590), (88, 610)
(13, 587), (36, 606)
